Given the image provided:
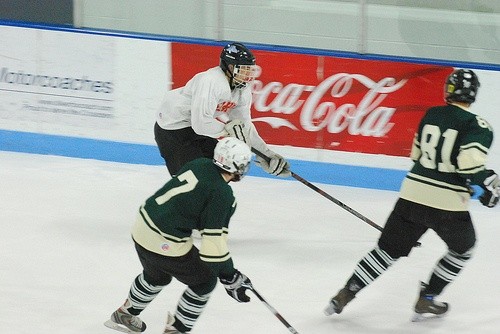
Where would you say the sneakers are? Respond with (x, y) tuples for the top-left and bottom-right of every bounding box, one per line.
(323, 286), (356, 316)
(159, 312), (194, 333)
(104, 305), (146, 333)
(411, 280), (448, 322)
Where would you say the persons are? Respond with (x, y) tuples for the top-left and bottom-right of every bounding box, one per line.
(323, 68), (500, 321)
(154, 43), (292, 179)
(105, 137), (254, 334)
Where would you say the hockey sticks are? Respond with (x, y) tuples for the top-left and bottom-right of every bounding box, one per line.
(248, 288), (299, 334)
(251, 146), (422, 247)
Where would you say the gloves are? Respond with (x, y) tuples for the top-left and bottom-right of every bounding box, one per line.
(484, 168), (500, 185)
(478, 184), (499, 207)
(219, 267), (253, 303)
(255, 148), (292, 178)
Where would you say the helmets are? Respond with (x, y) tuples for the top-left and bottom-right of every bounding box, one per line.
(221, 41), (255, 88)
(212, 136), (252, 181)
(444, 66), (480, 106)
